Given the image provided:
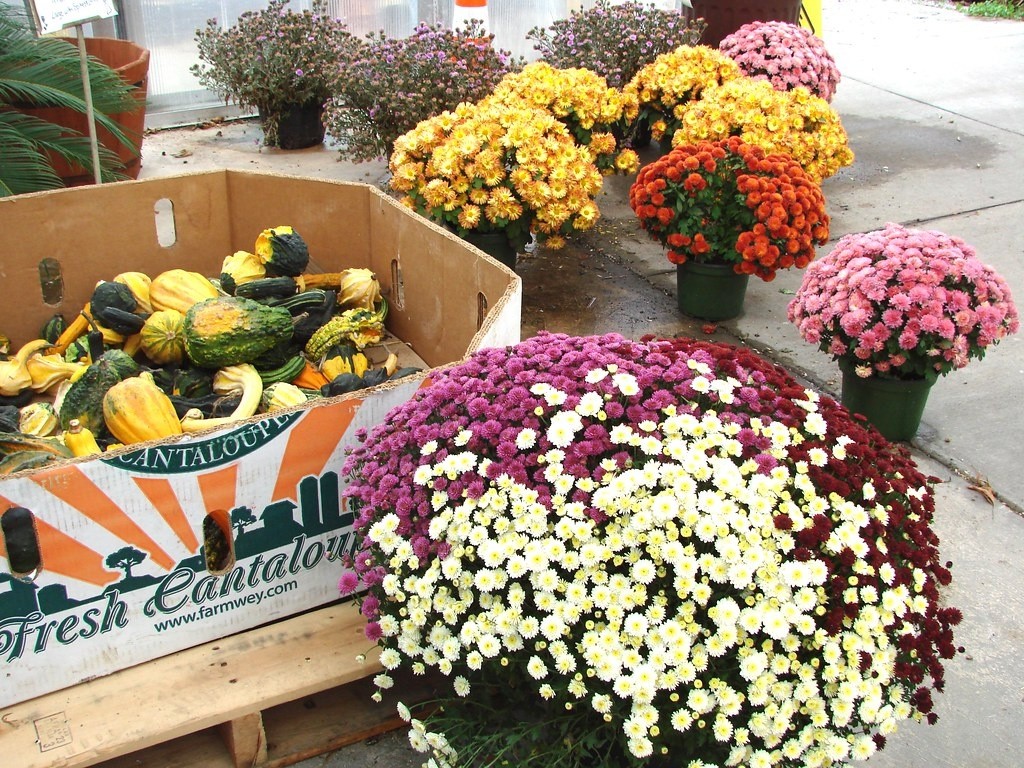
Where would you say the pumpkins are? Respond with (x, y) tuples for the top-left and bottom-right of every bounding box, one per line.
(0, 226), (426, 574)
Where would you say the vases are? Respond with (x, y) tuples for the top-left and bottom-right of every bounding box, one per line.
(444, 222), (517, 272)
(677, 260), (749, 321)
(257, 88), (332, 150)
(610, 106), (652, 151)
(842, 362), (941, 443)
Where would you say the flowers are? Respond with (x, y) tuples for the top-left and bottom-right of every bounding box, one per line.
(191, 0), (855, 281)
(345, 332), (962, 768)
(786, 221), (1020, 384)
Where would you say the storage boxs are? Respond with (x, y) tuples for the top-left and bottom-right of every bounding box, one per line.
(0, 170), (523, 710)
